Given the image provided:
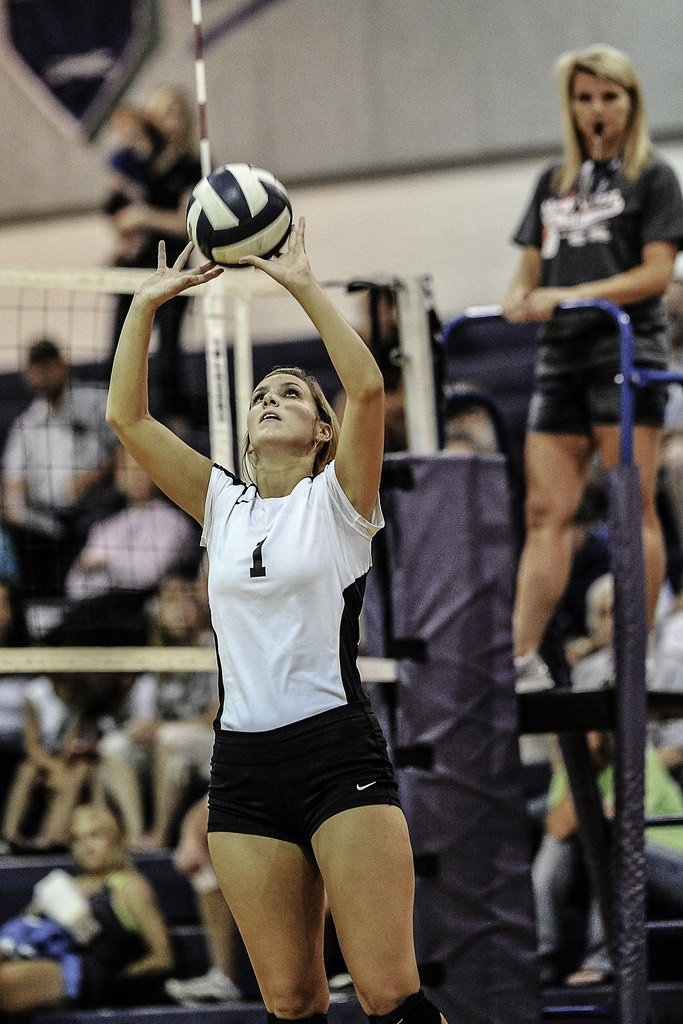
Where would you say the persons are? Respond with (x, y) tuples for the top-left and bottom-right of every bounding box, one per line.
(499, 43), (682, 698)
(104, 88), (200, 363)
(0, 338), (205, 646)
(481, 571), (680, 1024)
(343, 275), (449, 451)
(0, 572), (213, 1012)
(106, 215), (446, 1024)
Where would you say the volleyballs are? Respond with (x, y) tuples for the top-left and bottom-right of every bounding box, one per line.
(183, 161), (297, 270)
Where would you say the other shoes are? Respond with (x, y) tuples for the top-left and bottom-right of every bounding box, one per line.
(569, 967), (605, 985)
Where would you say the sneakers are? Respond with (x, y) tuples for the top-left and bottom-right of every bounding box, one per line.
(166, 969), (241, 1001)
(512, 650), (556, 693)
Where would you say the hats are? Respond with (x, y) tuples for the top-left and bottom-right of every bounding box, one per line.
(28, 341), (61, 365)
